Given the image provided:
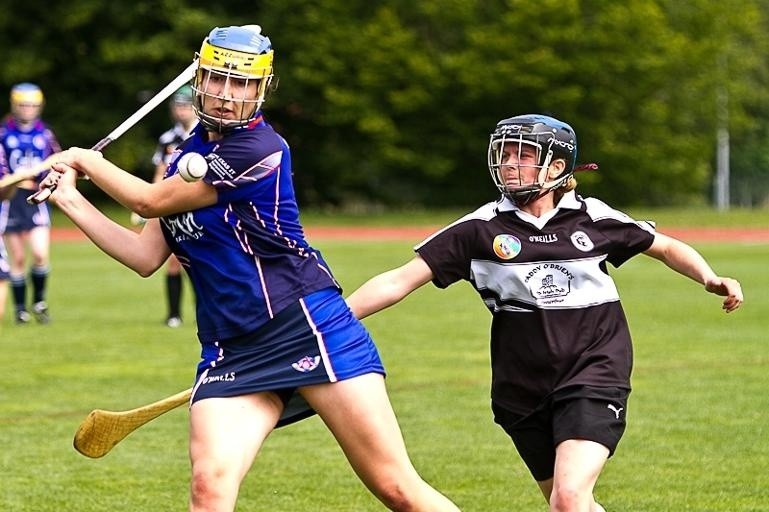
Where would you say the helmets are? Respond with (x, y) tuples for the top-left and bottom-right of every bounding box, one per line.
(492, 113), (578, 180)
(191, 24), (275, 81)
(9, 82), (47, 113)
(170, 83), (193, 103)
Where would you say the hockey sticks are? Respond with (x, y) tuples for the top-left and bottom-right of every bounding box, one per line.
(73, 387), (192, 459)
(30, 58), (199, 204)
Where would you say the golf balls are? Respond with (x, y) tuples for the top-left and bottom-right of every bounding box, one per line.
(177, 150), (208, 183)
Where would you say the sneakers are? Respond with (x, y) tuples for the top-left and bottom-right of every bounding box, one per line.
(166, 317), (182, 328)
(17, 301), (52, 322)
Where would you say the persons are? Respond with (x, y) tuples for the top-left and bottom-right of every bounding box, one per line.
(153, 82), (199, 329)
(345, 115), (744, 512)
(39, 27), (461, 512)
(1, 82), (62, 324)
